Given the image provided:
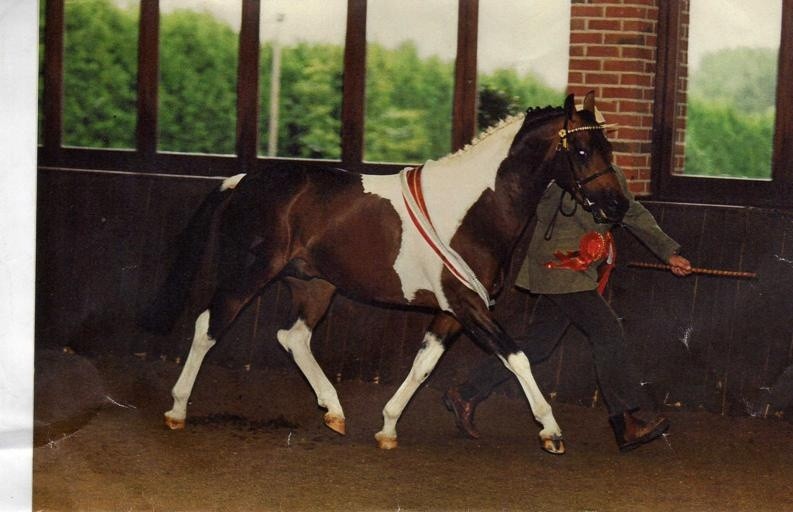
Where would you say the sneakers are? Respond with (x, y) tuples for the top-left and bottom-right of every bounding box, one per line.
(444, 384), (484, 438)
(608, 410), (671, 452)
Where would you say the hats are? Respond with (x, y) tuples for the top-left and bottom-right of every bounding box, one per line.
(573, 101), (620, 133)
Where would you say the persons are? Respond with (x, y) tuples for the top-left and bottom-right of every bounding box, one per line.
(442, 104), (692, 453)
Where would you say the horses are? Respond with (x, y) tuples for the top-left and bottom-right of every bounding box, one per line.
(135, 85), (632, 458)
